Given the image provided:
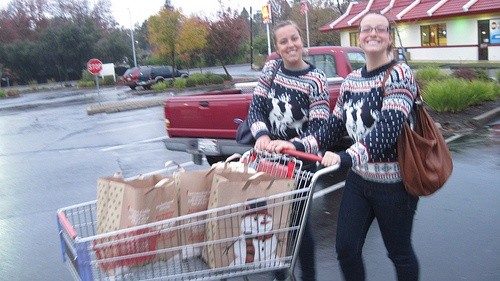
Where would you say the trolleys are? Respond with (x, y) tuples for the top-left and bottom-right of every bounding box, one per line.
(56, 148), (342, 281)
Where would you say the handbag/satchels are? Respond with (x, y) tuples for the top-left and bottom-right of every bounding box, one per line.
(233, 115), (255, 146)
(94, 153), (297, 277)
(381, 62), (453, 197)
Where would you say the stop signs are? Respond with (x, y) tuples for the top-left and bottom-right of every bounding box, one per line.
(87, 59), (102, 75)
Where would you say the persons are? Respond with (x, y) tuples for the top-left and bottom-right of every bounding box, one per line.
(266, 11), (421, 280)
(248, 21), (331, 281)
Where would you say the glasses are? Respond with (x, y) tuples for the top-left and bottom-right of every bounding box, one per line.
(359, 25), (389, 34)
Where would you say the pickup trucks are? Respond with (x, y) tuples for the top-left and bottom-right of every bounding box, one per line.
(123, 65), (189, 91)
(162, 46), (366, 168)
